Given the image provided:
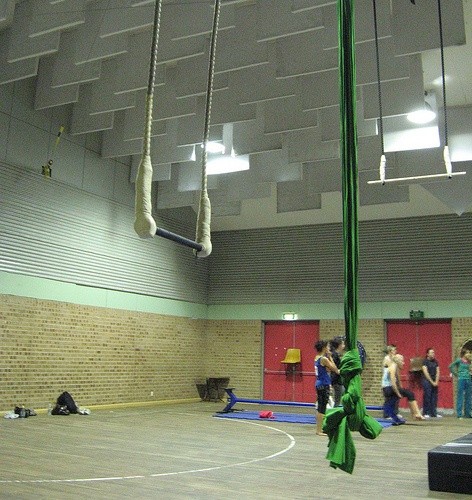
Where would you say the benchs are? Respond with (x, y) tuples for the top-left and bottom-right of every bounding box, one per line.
(427, 433), (472, 495)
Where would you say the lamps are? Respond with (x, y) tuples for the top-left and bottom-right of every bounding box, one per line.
(405, 100), (437, 125)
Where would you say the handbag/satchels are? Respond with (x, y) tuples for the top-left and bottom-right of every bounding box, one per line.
(58, 391), (78, 416)
(14, 406), (36, 417)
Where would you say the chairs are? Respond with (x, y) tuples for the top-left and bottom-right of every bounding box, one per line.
(280, 348), (301, 370)
(196, 378), (230, 403)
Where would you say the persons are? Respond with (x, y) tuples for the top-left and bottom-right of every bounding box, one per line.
(449, 347), (472, 419)
(422, 347), (442, 418)
(313, 338), (346, 436)
(381, 345), (427, 420)
